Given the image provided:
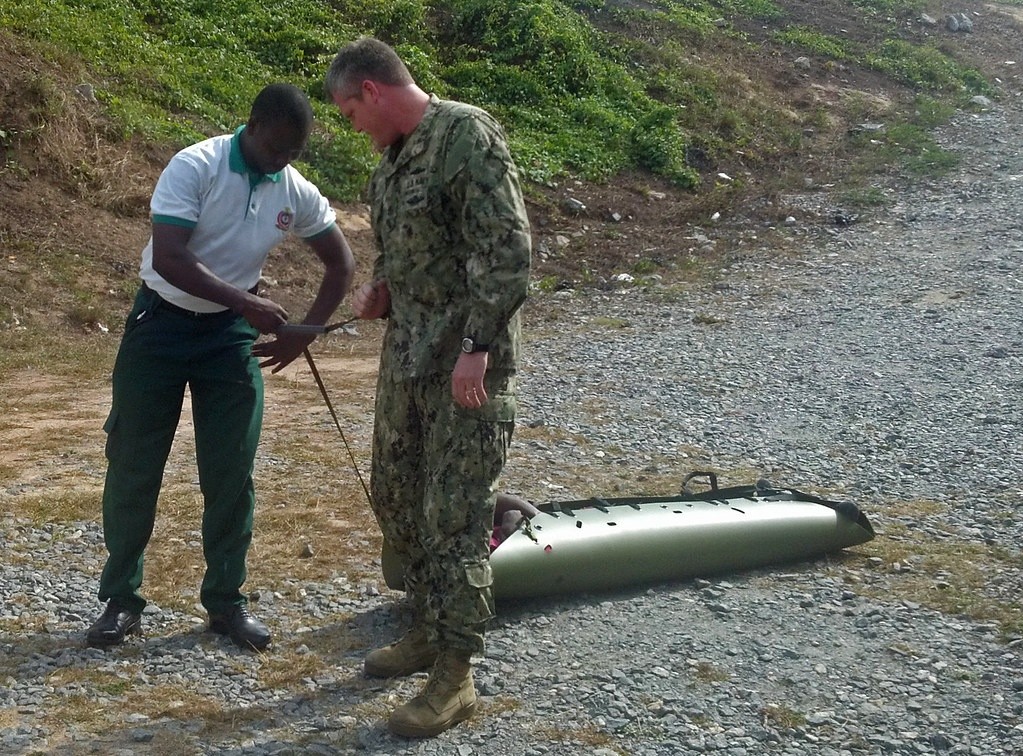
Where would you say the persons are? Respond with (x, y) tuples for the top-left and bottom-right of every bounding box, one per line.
(326, 36), (532, 738)
(86, 83), (353, 652)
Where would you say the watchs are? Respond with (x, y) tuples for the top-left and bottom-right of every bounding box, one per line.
(460, 337), (489, 353)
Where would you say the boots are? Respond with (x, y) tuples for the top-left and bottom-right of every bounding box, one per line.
(365, 622), (440, 678)
(390, 651), (478, 738)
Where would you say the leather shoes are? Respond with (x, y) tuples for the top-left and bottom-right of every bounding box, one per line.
(208, 603), (270, 646)
(84, 598), (142, 648)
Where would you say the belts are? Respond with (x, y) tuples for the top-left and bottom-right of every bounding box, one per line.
(143, 281), (221, 317)
